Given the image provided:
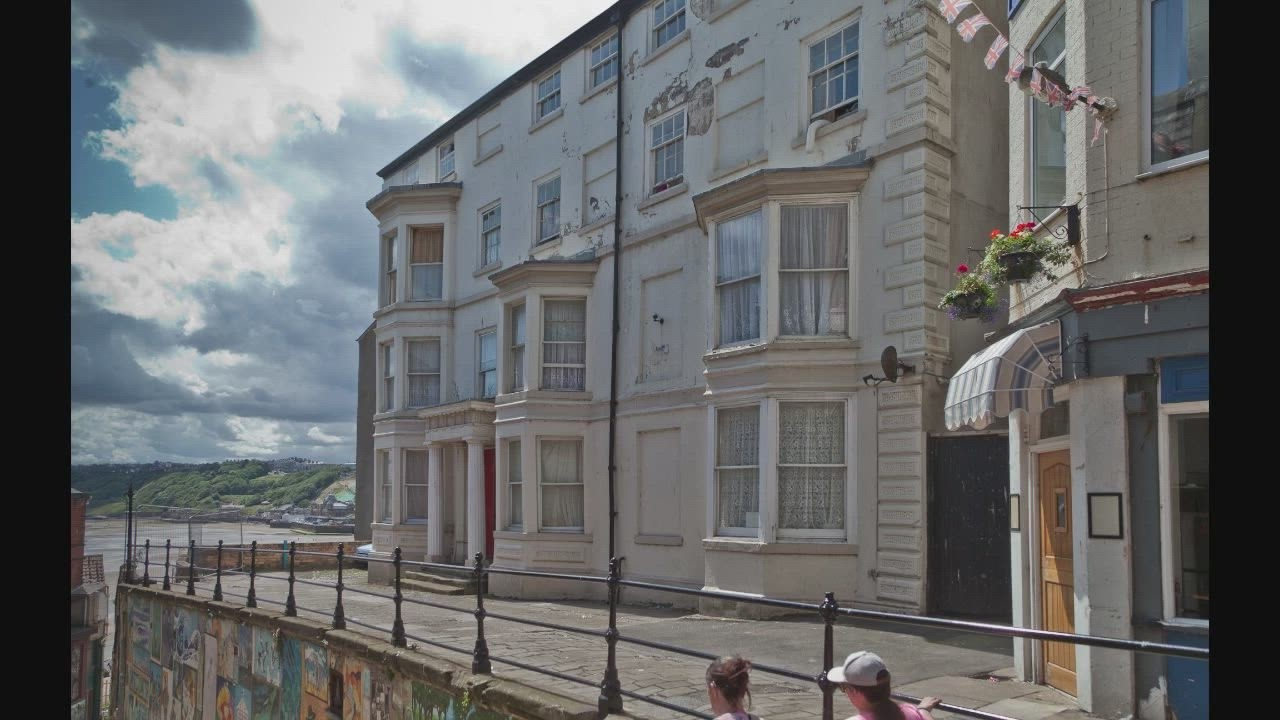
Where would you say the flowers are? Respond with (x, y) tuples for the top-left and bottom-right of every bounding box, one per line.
(937, 221), (1071, 323)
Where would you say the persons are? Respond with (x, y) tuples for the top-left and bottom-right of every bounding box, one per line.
(826, 650), (942, 720)
(705, 653), (760, 720)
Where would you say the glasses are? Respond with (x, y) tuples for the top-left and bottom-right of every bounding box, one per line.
(838, 682), (857, 693)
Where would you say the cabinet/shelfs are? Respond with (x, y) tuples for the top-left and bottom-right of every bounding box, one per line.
(1179, 485), (1211, 620)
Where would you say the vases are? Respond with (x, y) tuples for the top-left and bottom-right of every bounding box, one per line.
(955, 294), (981, 320)
(998, 252), (1033, 284)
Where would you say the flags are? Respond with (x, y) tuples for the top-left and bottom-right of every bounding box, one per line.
(1028, 61), (1099, 112)
(983, 33), (1008, 71)
(956, 12), (991, 43)
(1004, 53), (1025, 84)
(938, 0), (972, 25)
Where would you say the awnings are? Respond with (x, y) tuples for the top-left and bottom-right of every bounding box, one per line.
(943, 318), (1061, 431)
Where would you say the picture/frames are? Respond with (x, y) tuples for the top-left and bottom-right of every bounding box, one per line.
(1087, 492), (1124, 540)
(1009, 494), (1020, 531)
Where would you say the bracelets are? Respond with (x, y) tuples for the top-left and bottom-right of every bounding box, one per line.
(918, 707), (928, 711)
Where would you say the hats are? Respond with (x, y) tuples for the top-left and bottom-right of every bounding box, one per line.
(826, 650), (892, 688)
